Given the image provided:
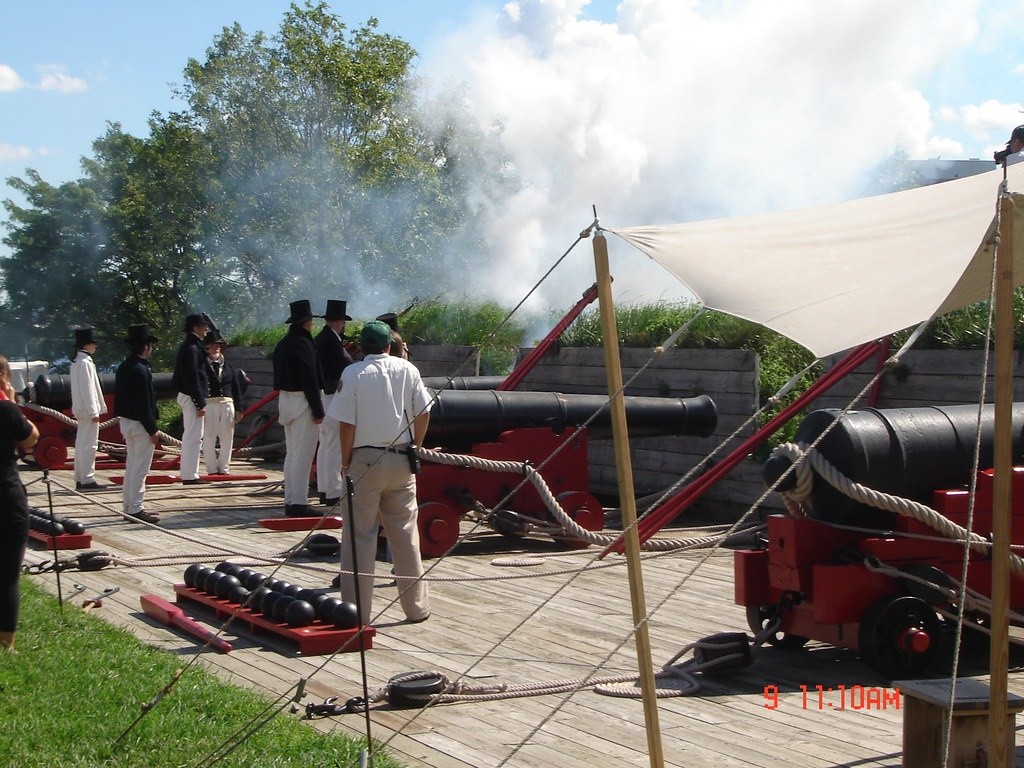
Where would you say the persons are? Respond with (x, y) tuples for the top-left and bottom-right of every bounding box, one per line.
(0, 355), (39, 651)
(70, 326), (108, 493)
(173, 314), (210, 484)
(114, 324), (162, 523)
(326, 321), (435, 623)
(998, 125), (1024, 179)
(271, 299), (326, 517)
(203, 331), (243, 476)
(313, 300), (352, 507)
(375, 312), (410, 360)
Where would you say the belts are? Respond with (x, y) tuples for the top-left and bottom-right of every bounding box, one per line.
(353, 446), (408, 455)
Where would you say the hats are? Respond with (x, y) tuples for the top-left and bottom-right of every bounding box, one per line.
(360, 320), (396, 347)
(204, 331), (224, 344)
(320, 300), (352, 321)
(73, 326), (97, 348)
(285, 300), (319, 324)
(123, 323), (159, 345)
(181, 313), (210, 331)
(376, 313), (397, 330)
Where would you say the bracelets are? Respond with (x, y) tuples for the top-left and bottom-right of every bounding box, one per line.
(341, 464), (349, 468)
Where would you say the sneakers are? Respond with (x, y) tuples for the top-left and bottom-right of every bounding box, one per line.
(124, 510), (159, 524)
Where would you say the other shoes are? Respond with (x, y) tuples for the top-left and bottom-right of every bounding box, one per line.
(319, 495), (340, 506)
(285, 503), (324, 517)
(410, 614), (429, 624)
(333, 574), (341, 589)
(183, 478), (211, 485)
(77, 482), (108, 493)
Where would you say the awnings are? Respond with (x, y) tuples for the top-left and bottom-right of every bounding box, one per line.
(592, 163), (1023, 768)
